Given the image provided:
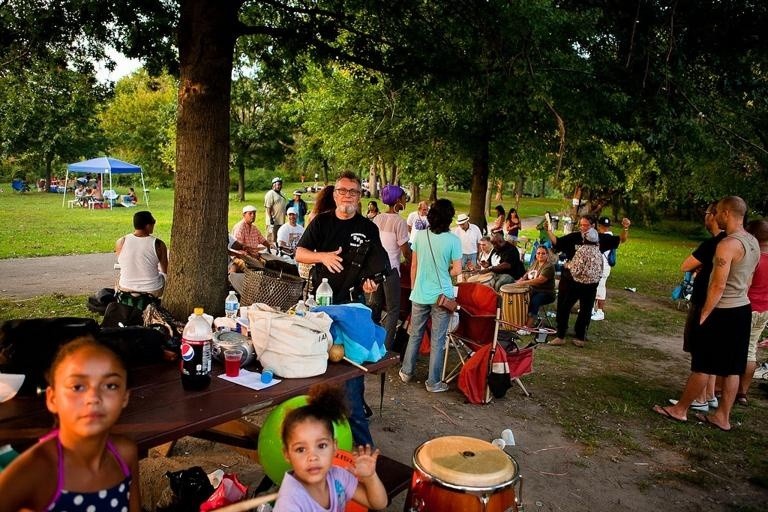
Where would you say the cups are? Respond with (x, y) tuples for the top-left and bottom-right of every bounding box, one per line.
(224, 349), (243, 377)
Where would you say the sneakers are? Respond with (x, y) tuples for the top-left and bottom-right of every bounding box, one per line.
(399, 368), (412, 383)
(516, 326), (531, 335)
(708, 398), (718, 408)
(425, 380), (450, 392)
(669, 399), (710, 412)
(591, 311), (604, 321)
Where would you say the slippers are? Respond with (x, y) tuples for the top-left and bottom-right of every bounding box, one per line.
(696, 412), (732, 432)
(650, 404), (687, 423)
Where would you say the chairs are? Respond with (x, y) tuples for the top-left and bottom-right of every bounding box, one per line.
(11, 178), (30, 194)
(535, 264), (564, 328)
(441, 282), (539, 403)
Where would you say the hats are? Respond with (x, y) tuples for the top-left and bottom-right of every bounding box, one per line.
(286, 207), (297, 216)
(584, 229), (599, 242)
(272, 177), (282, 183)
(381, 186), (402, 204)
(292, 190), (302, 196)
(455, 214), (469, 225)
(243, 206), (256, 213)
(598, 217), (613, 226)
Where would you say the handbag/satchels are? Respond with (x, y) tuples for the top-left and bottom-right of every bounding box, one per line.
(391, 314), (431, 358)
(100, 303), (143, 327)
(142, 302), (186, 365)
(437, 293), (457, 314)
(249, 302), (334, 378)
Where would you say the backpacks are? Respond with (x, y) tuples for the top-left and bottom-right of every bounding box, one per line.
(608, 249), (616, 267)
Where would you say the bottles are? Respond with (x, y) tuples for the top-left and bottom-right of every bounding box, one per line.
(316, 278), (334, 306)
(306, 295), (316, 308)
(181, 308), (212, 390)
(296, 300), (306, 316)
(225, 290), (239, 318)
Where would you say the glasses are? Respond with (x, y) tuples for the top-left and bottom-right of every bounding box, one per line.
(479, 244), (486, 246)
(536, 252), (546, 255)
(336, 188), (360, 196)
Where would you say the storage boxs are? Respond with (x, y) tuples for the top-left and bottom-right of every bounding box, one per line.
(49, 185), (58, 193)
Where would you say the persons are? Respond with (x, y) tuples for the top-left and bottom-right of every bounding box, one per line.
(542, 215), (631, 348)
(85, 184), (105, 208)
(650, 194), (768, 430)
(228, 174), (338, 282)
(73, 184), (94, 208)
(293, 170), (391, 450)
(512, 246), (557, 335)
(95, 174), (103, 194)
(1, 334), (143, 512)
(270, 379), (390, 512)
(122, 187), (138, 206)
(590, 216), (616, 322)
(534, 210), (557, 250)
(397, 197), (463, 394)
(361, 187), (526, 335)
(115, 210), (169, 298)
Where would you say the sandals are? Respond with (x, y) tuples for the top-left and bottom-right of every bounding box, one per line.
(716, 391), (721, 398)
(737, 394), (749, 407)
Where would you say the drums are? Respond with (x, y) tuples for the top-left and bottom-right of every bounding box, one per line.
(404, 436), (523, 512)
(500, 284), (530, 331)
(468, 273), (495, 289)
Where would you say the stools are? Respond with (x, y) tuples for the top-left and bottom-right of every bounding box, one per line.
(143, 189), (150, 202)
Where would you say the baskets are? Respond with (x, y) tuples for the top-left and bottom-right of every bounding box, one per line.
(240, 265), (306, 312)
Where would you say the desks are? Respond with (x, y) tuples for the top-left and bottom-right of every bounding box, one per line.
(0, 349), (414, 511)
(75, 195), (95, 208)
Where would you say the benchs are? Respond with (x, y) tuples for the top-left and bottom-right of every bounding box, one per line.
(68, 199), (79, 208)
(375, 453), (416, 500)
(89, 200), (104, 209)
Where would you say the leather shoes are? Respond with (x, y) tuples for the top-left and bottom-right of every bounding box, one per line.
(572, 340), (585, 348)
(547, 341), (565, 346)
